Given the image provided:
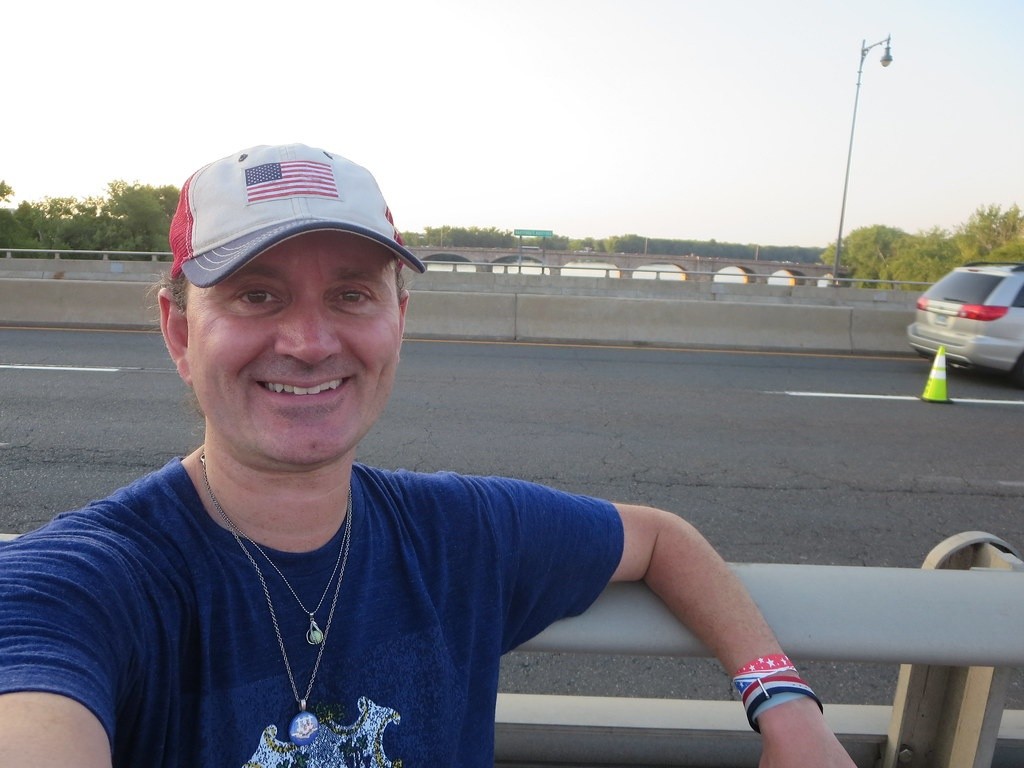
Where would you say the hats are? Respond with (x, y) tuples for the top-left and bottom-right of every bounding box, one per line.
(167, 144), (427, 295)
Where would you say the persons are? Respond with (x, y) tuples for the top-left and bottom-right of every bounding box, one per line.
(0, 144), (857, 768)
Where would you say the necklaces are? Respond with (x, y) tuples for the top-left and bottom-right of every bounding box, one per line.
(198, 447), (353, 747)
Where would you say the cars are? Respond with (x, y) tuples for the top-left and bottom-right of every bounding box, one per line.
(903, 260), (1024, 390)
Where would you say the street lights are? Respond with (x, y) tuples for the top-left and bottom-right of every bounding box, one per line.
(830, 35), (893, 287)
(919, 346), (953, 406)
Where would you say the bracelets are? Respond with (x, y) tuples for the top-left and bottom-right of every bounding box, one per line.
(742, 675), (824, 734)
